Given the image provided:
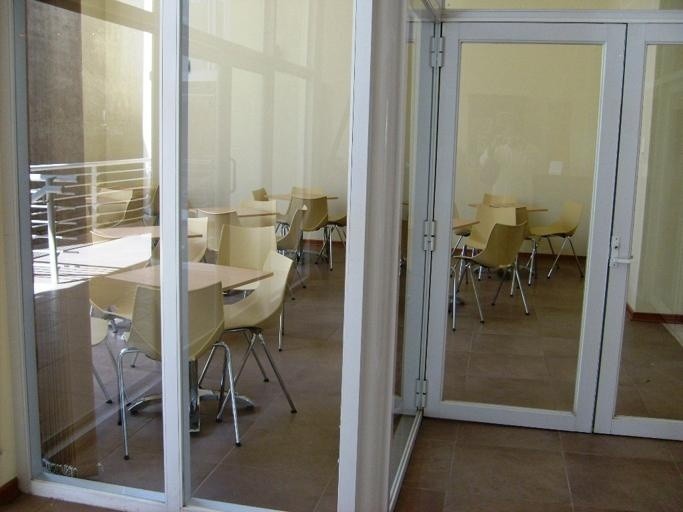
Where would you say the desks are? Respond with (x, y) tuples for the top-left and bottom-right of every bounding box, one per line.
(111, 261), (275, 432)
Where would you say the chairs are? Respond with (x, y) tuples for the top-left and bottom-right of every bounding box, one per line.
(88, 314), (129, 409)
(116, 280), (242, 460)
(70, 186), (349, 356)
(224, 253), (298, 414)
(402, 176), (583, 333)
